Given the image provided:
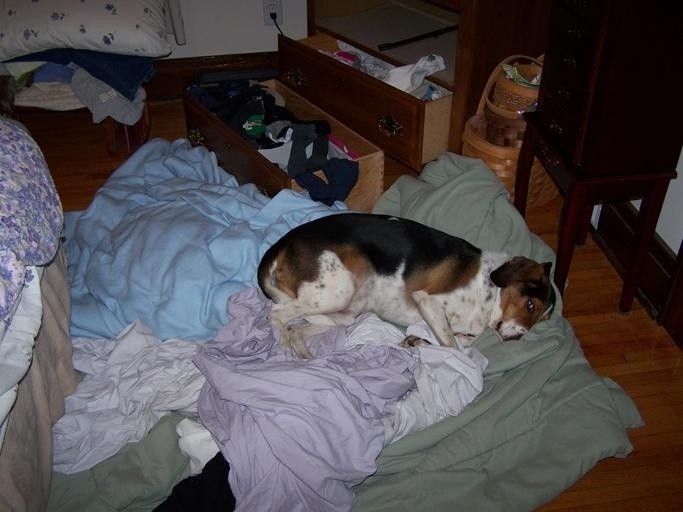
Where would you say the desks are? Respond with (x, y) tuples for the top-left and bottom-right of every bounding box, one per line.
(512, 111), (679, 316)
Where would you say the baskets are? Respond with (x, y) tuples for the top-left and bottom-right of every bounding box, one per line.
(461, 55), (559, 210)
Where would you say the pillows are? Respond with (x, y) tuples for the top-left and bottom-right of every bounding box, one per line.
(0, 0), (176, 65)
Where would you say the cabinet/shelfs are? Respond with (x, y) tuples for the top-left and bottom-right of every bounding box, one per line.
(535, 1), (682, 177)
(274, 0), (551, 178)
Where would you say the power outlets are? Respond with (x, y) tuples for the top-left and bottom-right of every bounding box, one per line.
(263, 0), (283, 26)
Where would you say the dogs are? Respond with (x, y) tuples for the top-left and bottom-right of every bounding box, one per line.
(255, 212), (557, 362)
